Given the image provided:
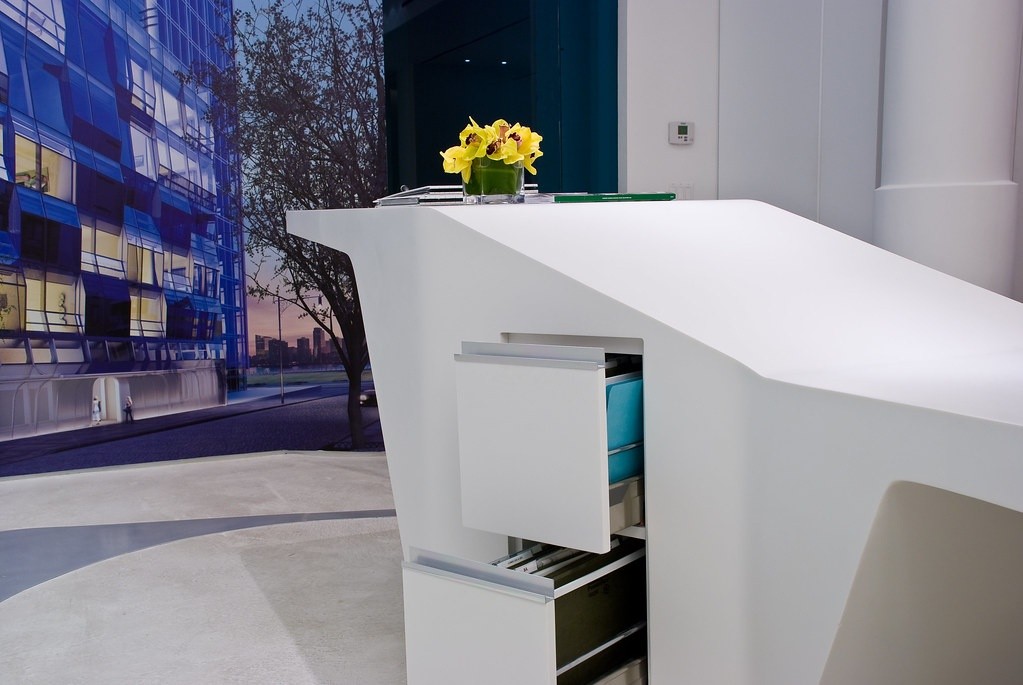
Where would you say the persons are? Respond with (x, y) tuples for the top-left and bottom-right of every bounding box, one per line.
(92, 396), (101, 422)
(123, 396), (134, 423)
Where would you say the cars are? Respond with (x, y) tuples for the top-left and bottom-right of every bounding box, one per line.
(359, 389), (378, 408)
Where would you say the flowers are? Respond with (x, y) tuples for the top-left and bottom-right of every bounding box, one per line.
(439, 114), (544, 184)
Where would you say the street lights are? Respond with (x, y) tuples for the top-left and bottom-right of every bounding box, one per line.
(274, 294), (322, 406)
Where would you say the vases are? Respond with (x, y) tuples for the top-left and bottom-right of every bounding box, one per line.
(462, 156), (520, 195)
